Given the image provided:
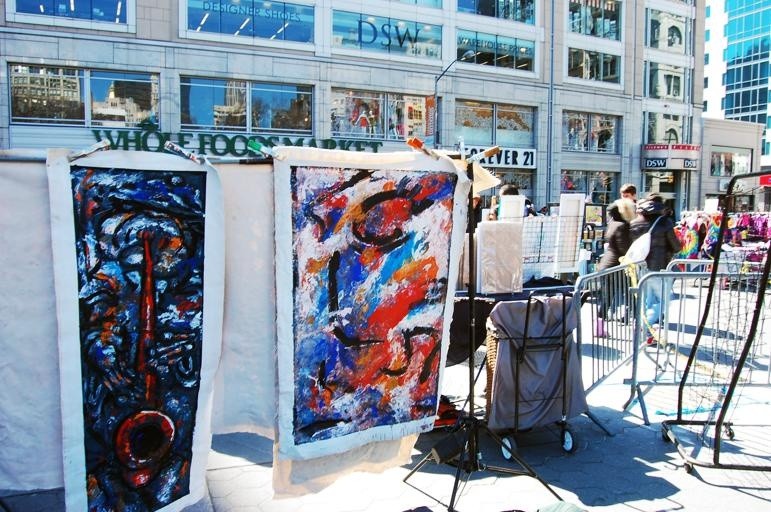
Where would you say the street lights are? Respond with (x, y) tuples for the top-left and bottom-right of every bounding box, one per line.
(434, 50), (475, 150)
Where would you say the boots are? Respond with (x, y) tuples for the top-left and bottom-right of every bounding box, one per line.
(595, 318), (609, 337)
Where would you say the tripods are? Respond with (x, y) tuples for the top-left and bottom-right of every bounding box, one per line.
(402, 163), (565, 512)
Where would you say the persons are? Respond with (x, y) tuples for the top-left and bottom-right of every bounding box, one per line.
(351, 97), (380, 139)
(593, 184), (771, 354)
(466, 184), (549, 233)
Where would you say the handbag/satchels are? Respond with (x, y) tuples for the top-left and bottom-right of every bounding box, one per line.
(620, 232), (651, 265)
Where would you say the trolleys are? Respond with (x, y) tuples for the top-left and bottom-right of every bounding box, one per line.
(485, 286), (580, 463)
